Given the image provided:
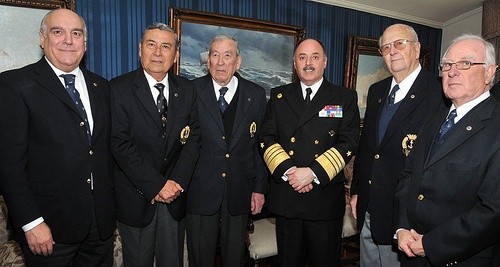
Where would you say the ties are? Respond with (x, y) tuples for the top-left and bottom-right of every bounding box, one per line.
(305, 88), (312, 101)
(155, 83), (168, 140)
(438, 109), (456, 141)
(219, 87), (230, 112)
(387, 85), (399, 105)
(60, 74), (92, 147)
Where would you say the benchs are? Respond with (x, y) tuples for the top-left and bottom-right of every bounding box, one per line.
(245, 195), (360, 267)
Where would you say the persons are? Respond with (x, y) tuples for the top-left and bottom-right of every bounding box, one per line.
(182, 35), (269, 267)
(392, 34), (500, 267)
(349, 23), (443, 267)
(0, 9), (117, 267)
(107, 22), (202, 267)
(258, 37), (360, 267)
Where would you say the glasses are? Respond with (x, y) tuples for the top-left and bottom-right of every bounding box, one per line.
(440, 61), (485, 72)
(381, 39), (412, 55)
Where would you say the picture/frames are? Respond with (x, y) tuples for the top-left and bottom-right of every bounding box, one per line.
(0, 0), (76, 73)
(345, 36), (432, 127)
(168, 6), (307, 83)
